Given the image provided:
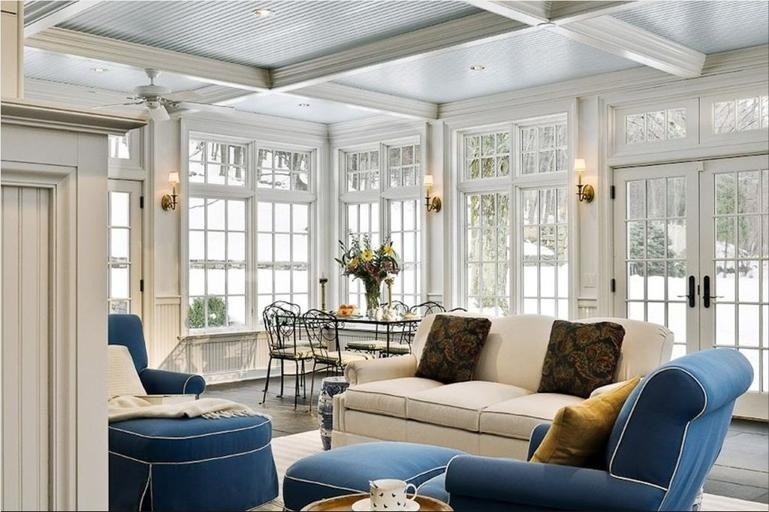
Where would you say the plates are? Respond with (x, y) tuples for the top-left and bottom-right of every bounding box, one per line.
(351, 498), (421, 511)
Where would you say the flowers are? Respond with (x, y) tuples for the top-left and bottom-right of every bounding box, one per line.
(334, 229), (404, 319)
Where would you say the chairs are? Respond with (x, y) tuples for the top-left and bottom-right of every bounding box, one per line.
(263, 300), (327, 399)
(344, 300), (410, 360)
(262, 305), (328, 409)
(379, 301), (447, 359)
(302, 309), (376, 413)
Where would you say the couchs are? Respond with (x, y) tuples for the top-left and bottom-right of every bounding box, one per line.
(110, 315), (279, 511)
(283, 349), (754, 511)
(327, 312), (675, 463)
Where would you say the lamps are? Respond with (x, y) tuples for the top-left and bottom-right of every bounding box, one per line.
(574, 158), (593, 203)
(162, 170), (179, 211)
(422, 174), (440, 213)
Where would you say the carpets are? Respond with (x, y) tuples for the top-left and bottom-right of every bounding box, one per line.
(244, 427), (769, 512)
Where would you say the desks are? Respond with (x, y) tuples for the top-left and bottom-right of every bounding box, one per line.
(278, 313), (423, 358)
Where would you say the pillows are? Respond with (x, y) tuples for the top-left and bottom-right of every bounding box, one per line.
(414, 316), (491, 384)
(537, 320), (625, 397)
(526, 374), (642, 466)
(109, 344), (147, 397)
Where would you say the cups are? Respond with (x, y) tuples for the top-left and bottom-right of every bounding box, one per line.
(369, 478), (419, 511)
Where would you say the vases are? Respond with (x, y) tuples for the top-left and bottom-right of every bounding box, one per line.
(365, 292), (381, 318)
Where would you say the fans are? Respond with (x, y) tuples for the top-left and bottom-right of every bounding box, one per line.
(91, 68), (236, 124)
